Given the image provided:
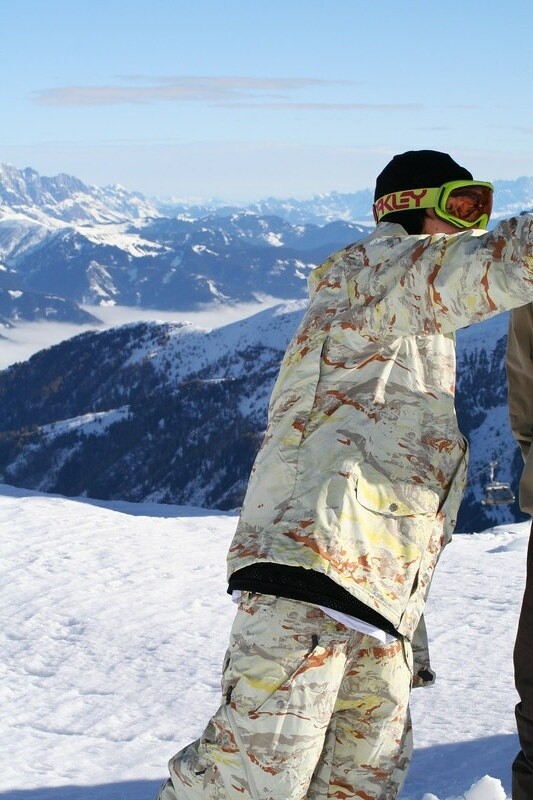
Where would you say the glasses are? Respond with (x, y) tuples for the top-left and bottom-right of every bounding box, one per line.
(436, 180), (494, 231)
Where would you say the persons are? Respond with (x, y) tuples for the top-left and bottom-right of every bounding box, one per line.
(503, 298), (533, 799)
(155, 149), (533, 800)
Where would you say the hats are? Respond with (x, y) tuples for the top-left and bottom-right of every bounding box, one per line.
(373, 151), (473, 227)
